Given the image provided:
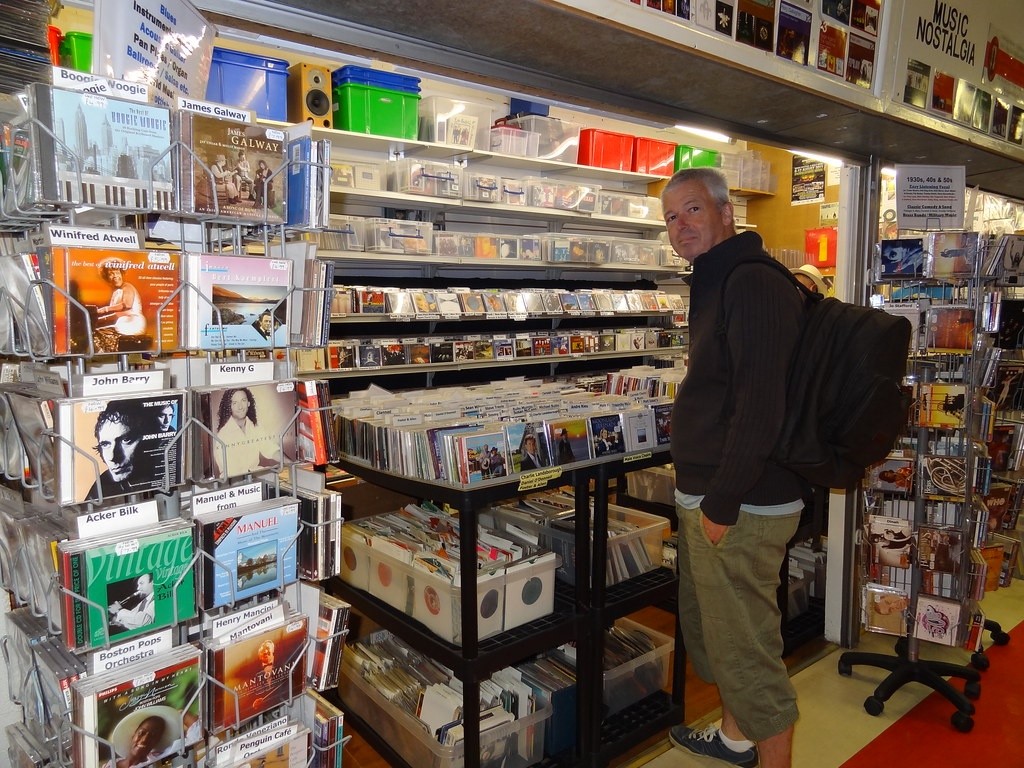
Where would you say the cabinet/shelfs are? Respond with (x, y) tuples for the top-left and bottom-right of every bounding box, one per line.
(251, 121), (776, 488)
(838, 229), (1024, 734)
(308, 452), (829, 767)
(0, 79), (353, 766)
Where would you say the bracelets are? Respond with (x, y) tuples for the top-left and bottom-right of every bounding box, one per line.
(105, 307), (108, 312)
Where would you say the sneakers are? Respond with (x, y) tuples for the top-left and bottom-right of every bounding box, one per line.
(669, 725), (760, 768)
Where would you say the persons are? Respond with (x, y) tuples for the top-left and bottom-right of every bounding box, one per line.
(879, 468), (910, 487)
(479, 444), (504, 478)
(101, 716), (165, 768)
(153, 402), (176, 432)
(210, 150), (273, 207)
(439, 237), (456, 255)
(214, 388), (295, 475)
(257, 313), (279, 338)
(599, 429), (613, 452)
(459, 237), (473, 256)
(254, 640), (287, 694)
(108, 573), (154, 633)
(661, 167), (805, 768)
(521, 435), (542, 470)
(96, 258), (147, 351)
(559, 428), (574, 458)
(86, 399), (153, 501)
(881, 239), (923, 270)
(873, 595), (909, 616)
(870, 529), (912, 550)
(454, 125), (469, 143)
(788, 265), (825, 295)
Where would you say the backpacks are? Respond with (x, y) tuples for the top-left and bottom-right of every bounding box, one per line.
(713, 255), (912, 490)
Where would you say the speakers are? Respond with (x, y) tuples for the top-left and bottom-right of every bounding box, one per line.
(288, 63), (333, 130)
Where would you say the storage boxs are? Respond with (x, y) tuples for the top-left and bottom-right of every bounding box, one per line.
(51, 23), (774, 267)
(326, 487), (816, 766)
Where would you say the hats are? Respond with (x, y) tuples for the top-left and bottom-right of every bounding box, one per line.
(788, 264), (828, 301)
